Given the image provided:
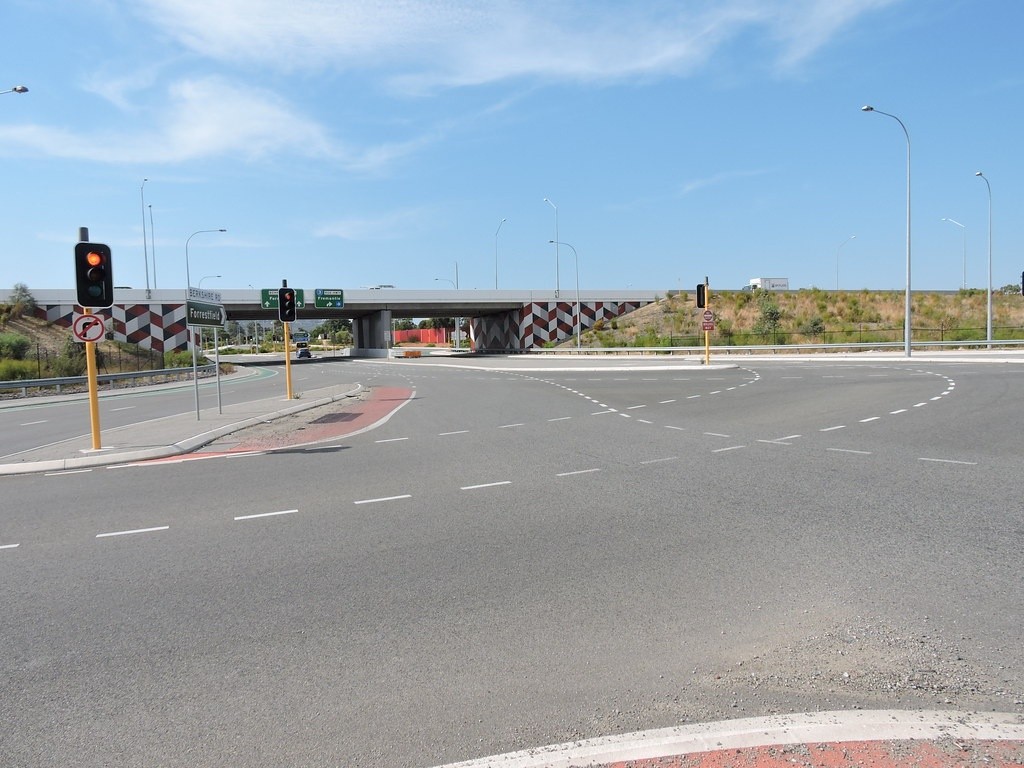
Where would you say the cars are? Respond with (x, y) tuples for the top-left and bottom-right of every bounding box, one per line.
(292, 333), (308, 342)
(296, 349), (311, 358)
(296, 342), (310, 351)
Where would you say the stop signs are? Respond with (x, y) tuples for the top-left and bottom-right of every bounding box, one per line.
(703, 310), (713, 321)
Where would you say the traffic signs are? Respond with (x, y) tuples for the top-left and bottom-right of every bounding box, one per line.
(315, 289), (344, 308)
(185, 300), (227, 328)
(261, 288), (305, 309)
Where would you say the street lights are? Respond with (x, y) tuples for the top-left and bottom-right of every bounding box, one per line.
(148, 205), (156, 289)
(942, 217), (966, 290)
(495, 219), (506, 289)
(550, 240), (581, 354)
(976, 172), (991, 349)
(862, 106), (912, 356)
(544, 198), (559, 290)
(836, 235), (858, 291)
(186, 230), (227, 421)
(140, 179), (149, 289)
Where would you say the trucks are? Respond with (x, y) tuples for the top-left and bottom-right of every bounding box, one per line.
(742, 277), (789, 291)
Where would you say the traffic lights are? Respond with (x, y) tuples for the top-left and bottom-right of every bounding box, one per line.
(74, 242), (114, 309)
(278, 289), (296, 323)
